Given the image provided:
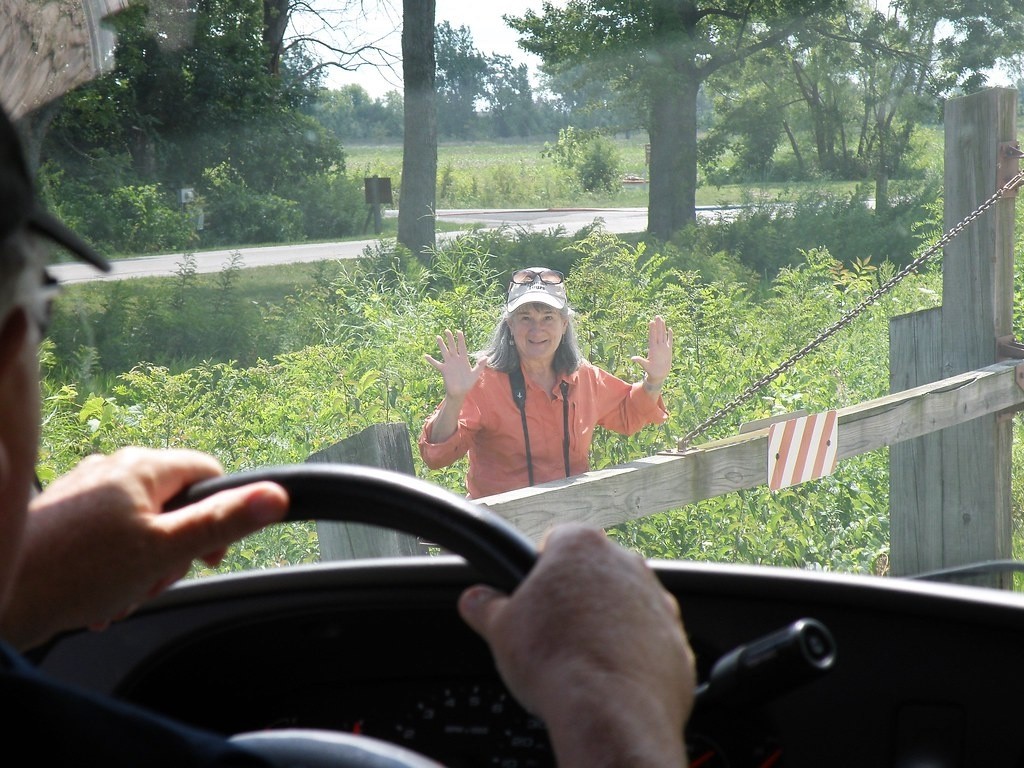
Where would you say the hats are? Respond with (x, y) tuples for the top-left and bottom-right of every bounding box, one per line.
(0, 106), (111, 272)
(506, 267), (567, 313)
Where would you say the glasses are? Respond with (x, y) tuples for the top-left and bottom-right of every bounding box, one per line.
(506, 270), (566, 300)
(23, 278), (56, 335)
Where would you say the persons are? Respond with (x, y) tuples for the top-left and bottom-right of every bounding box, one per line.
(0, 101), (690, 768)
(420, 267), (674, 500)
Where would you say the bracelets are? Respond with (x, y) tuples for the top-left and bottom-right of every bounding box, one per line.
(644, 376), (664, 391)
